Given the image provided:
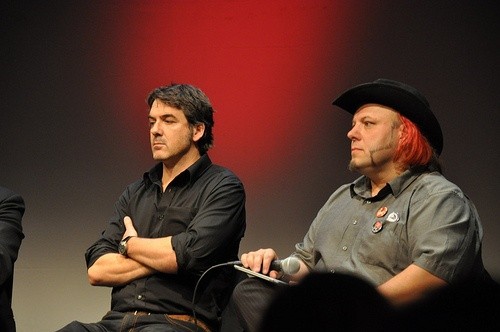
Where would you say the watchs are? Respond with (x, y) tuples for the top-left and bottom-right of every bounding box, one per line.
(118, 236), (133, 258)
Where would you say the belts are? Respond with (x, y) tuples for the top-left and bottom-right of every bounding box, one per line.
(124, 310), (212, 332)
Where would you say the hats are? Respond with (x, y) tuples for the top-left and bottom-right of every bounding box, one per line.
(331, 78), (443, 156)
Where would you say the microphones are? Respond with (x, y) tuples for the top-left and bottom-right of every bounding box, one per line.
(230, 257), (301, 275)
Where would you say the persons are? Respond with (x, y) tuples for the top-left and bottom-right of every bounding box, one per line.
(55, 83), (245, 332)
(0, 183), (25, 332)
(233, 79), (483, 332)
